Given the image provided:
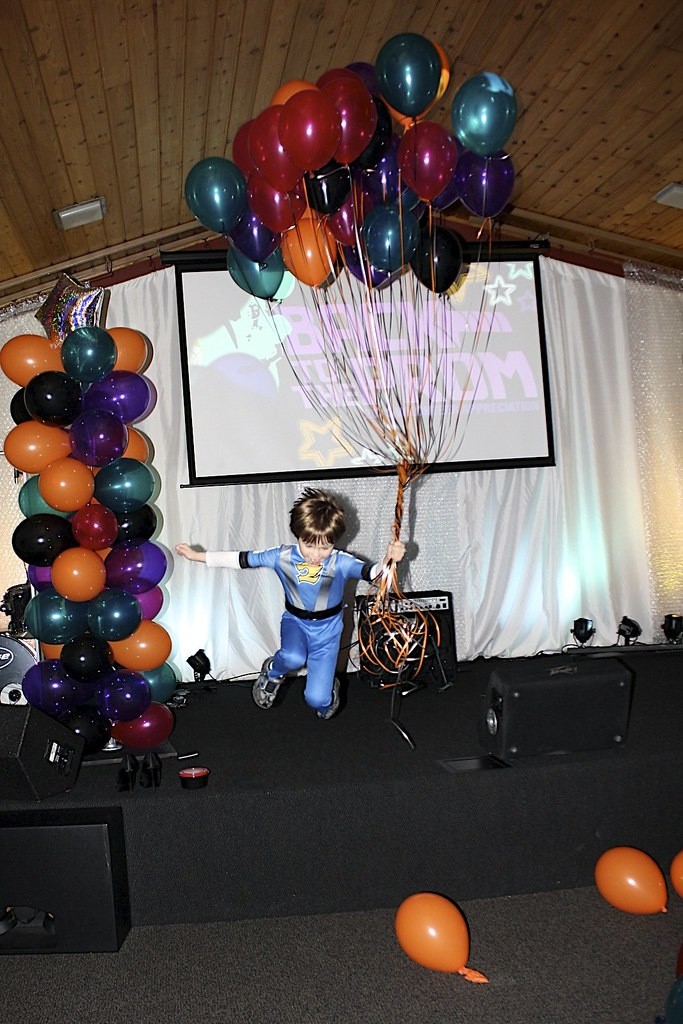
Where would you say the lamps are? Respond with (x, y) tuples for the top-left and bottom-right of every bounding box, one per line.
(187, 649), (213, 681)
(661, 614), (683, 644)
(570, 618), (597, 650)
(615, 616), (643, 647)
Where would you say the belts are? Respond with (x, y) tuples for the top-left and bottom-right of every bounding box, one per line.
(285, 596), (345, 620)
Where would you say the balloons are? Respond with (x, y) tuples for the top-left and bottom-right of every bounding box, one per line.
(453, 72), (515, 158)
(670, 852), (683, 897)
(376, 33), (440, 116)
(413, 230), (461, 294)
(396, 892), (470, 972)
(232, 41), (463, 289)
(0, 328), (178, 750)
(232, 209), (274, 262)
(594, 847), (667, 914)
(228, 249), (284, 297)
(184, 157), (245, 234)
(458, 151), (513, 217)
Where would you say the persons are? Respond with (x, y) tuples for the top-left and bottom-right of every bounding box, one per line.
(176, 491), (404, 719)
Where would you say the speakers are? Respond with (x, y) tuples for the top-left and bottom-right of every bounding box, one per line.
(355, 589), (457, 687)
(0, 806), (132, 954)
(0, 703), (86, 801)
(478, 655), (632, 761)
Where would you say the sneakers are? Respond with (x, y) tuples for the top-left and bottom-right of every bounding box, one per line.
(317, 676), (340, 720)
(252, 656), (287, 710)
